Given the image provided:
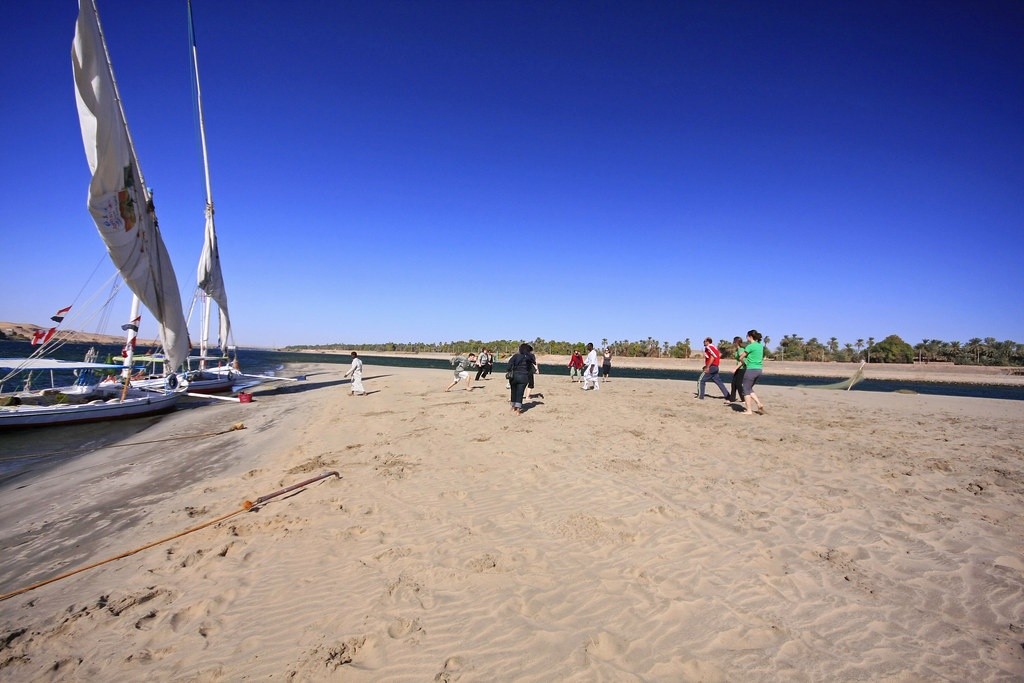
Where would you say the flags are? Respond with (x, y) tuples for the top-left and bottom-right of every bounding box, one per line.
(121, 336), (136, 358)
(31, 327), (56, 346)
(51, 305), (73, 324)
(121, 315), (141, 333)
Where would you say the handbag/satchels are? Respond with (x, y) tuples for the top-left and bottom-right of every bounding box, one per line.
(506, 369), (513, 379)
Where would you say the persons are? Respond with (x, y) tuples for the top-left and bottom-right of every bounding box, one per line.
(694, 337), (731, 401)
(568, 343), (599, 391)
(445, 347), (494, 392)
(343, 352), (367, 397)
(724, 330), (764, 415)
(505, 344), (539, 416)
(601, 348), (611, 382)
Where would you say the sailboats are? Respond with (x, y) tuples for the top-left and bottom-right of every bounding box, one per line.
(97, 0), (248, 393)
(0, 0), (193, 428)
